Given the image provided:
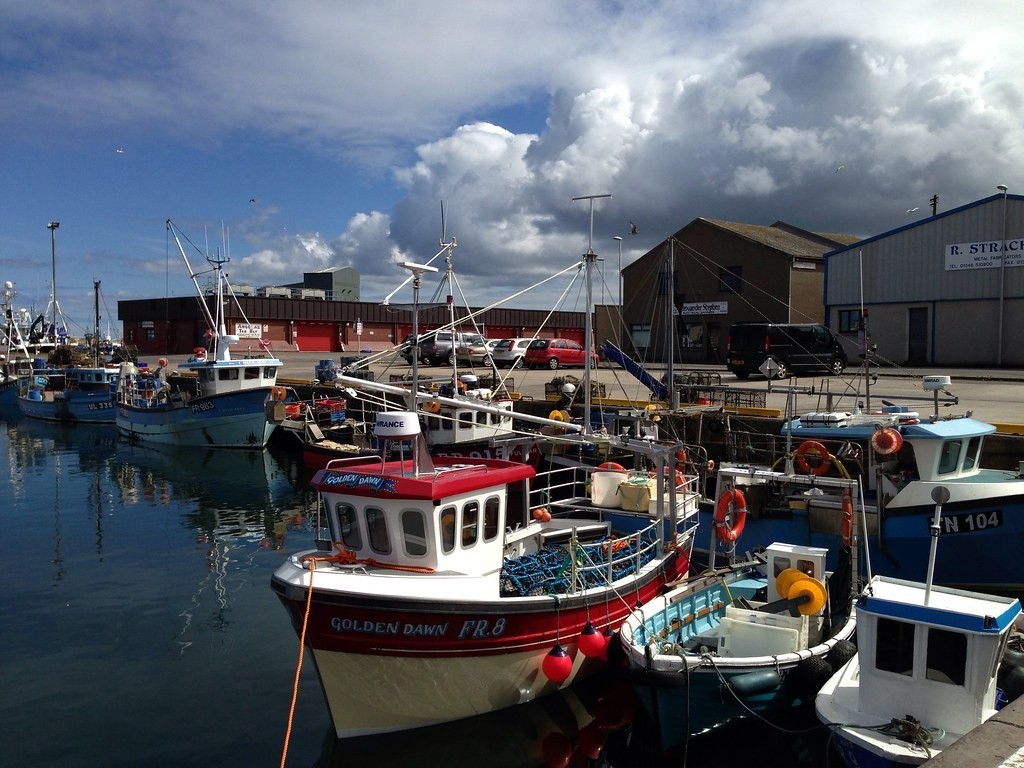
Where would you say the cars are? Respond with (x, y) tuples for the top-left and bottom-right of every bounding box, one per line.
(492, 338), (541, 370)
(525, 338), (601, 371)
(456, 338), (503, 367)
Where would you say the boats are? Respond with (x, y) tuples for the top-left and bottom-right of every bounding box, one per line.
(112, 218), (295, 452)
(243, 190), (1024, 738)
(815, 484), (1024, 768)
(1, 280), (138, 427)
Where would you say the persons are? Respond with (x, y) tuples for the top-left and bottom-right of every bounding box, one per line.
(452, 371), (461, 386)
(562, 383), (575, 397)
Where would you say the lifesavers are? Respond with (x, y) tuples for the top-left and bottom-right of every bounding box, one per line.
(796, 440), (831, 475)
(592, 461), (632, 481)
(715, 489), (747, 544)
(647, 465), (688, 495)
(840, 486), (854, 548)
(657, 439), (686, 475)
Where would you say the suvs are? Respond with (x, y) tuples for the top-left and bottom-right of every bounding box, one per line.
(401, 333), (422, 365)
(421, 330), (484, 367)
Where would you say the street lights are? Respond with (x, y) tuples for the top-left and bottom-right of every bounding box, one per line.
(47, 221), (60, 339)
(611, 235), (623, 351)
(996, 183), (1007, 369)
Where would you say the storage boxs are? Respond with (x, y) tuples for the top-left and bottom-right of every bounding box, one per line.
(314, 399), (345, 422)
(619, 478), (659, 511)
(718, 597), (809, 658)
(648, 492), (696, 517)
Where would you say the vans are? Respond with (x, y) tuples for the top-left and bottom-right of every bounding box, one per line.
(723, 322), (847, 382)
